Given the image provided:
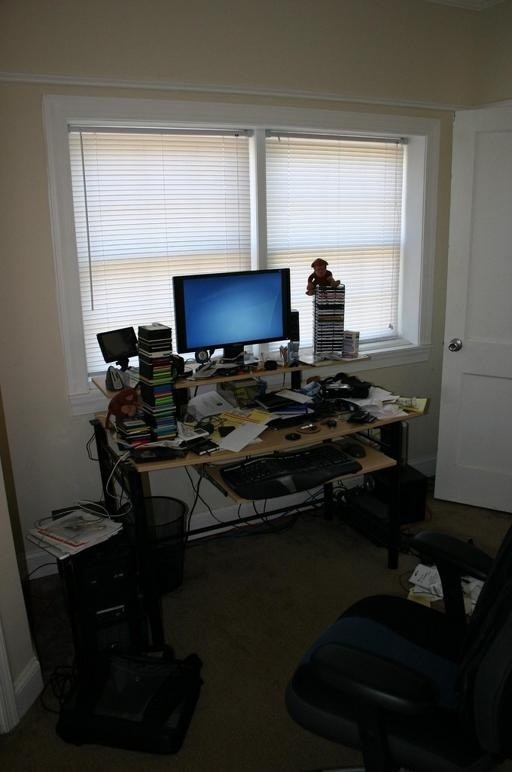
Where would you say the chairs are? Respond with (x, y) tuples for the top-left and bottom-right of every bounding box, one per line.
(285, 521), (512, 772)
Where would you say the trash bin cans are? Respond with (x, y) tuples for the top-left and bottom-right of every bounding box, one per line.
(146, 494), (188, 585)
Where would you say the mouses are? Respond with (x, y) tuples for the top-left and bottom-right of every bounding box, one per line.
(343, 444), (366, 458)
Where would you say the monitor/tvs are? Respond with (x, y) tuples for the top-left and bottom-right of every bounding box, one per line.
(96, 327), (141, 373)
(172, 268), (290, 367)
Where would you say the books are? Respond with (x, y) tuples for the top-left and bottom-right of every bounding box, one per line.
(176, 391), (274, 451)
(134, 323), (179, 442)
(408, 562), (486, 617)
(117, 418), (151, 440)
(343, 331), (360, 357)
(24, 510), (124, 560)
(311, 284), (345, 355)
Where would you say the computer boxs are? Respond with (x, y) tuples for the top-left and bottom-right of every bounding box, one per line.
(48, 498), (146, 665)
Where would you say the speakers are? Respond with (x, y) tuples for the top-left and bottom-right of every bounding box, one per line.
(195, 351), (208, 364)
(363, 463), (426, 528)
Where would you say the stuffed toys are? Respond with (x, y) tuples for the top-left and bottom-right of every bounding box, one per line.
(305, 258), (340, 296)
(106, 387), (138, 430)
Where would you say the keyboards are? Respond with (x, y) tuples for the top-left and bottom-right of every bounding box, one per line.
(219, 442), (363, 500)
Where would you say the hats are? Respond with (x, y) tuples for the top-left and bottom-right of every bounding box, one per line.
(312, 258), (328, 266)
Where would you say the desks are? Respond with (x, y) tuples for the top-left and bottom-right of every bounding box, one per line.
(90, 359), (425, 646)
